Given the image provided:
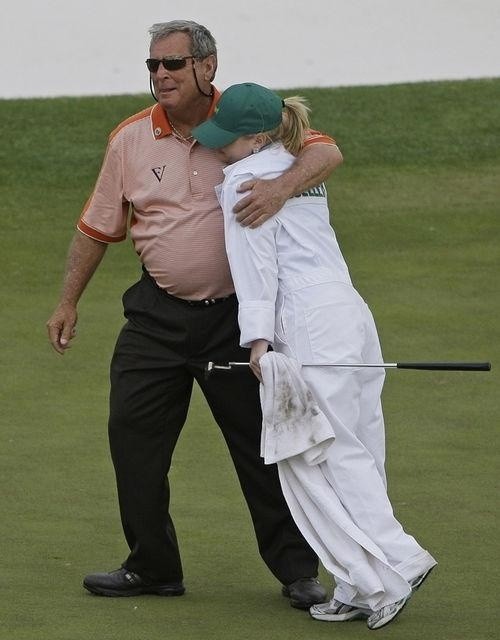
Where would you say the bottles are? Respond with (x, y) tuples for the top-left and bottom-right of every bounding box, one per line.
(191, 83), (283, 148)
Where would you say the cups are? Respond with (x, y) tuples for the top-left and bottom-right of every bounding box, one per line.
(146, 56), (196, 73)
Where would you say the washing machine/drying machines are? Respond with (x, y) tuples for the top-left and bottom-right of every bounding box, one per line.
(204, 363), (491, 371)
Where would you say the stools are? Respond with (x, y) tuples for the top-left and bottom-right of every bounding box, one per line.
(142, 265), (216, 306)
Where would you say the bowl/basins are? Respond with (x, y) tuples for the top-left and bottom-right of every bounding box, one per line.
(167, 118), (196, 140)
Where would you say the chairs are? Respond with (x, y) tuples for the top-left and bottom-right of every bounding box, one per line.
(83, 568), (185, 597)
(282, 578), (326, 609)
(309, 599), (372, 622)
(367, 562), (438, 629)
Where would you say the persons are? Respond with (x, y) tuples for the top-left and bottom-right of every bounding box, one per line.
(44, 18), (345, 611)
(189, 80), (440, 630)
